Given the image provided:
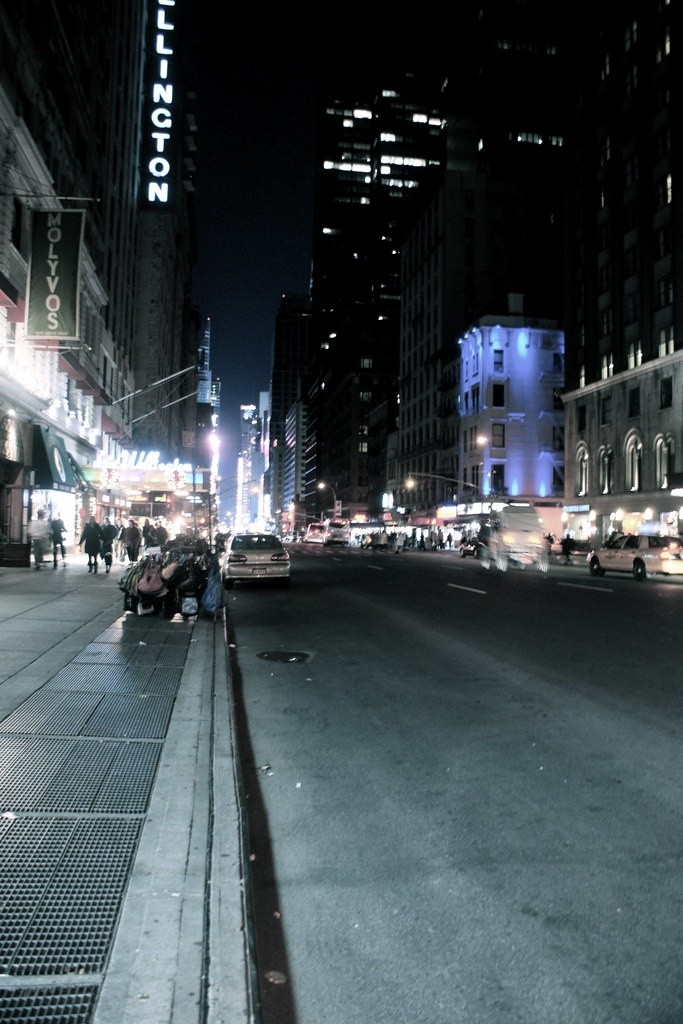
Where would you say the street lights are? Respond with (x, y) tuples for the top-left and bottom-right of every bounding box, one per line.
(319, 482), (336, 518)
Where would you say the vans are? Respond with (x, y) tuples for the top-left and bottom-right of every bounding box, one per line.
(321, 519), (351, 547)
(303, 523), (325, 544)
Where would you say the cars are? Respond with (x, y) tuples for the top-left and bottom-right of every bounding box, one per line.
(460, 540), (487, 559)
(478, 505), (551, 573)
(217, 534), (291, 591)
(586, 535), (683, 581)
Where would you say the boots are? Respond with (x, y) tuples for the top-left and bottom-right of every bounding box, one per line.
(88, 562), (92, 572)
(94, 562), (97, 573)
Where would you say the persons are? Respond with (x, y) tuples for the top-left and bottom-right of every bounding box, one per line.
(560, 534), (574, 564)
(545, 532), (554, 555)
(27, 511), (53, 568)
(79, 515), (168, 574)
(354, 529), (467, 553)
(50, 512), (68, 567)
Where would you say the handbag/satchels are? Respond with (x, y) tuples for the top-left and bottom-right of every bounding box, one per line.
(118, 550), (228, 623)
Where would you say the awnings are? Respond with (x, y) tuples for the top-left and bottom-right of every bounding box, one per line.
(68, 452), (97, 493)
(33, 424), (77, 494)
